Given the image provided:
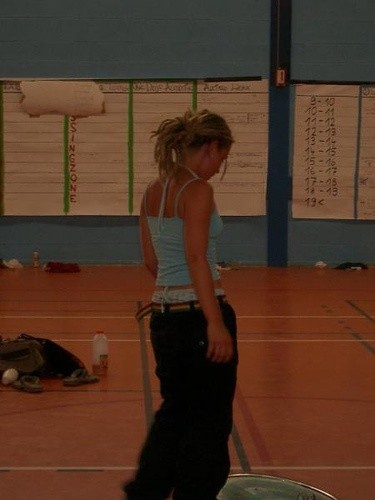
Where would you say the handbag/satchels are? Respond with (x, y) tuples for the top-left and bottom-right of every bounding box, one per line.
(0, 333), (84, 378)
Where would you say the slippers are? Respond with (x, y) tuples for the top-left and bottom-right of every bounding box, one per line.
(12, 375), (43, 392)
(62, 369), (99, 386)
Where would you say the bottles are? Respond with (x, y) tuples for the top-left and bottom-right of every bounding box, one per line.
(93, 330), (109, 368)
(32, 251), (39, 267)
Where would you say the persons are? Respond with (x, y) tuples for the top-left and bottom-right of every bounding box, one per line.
(123, 109), (239, 499)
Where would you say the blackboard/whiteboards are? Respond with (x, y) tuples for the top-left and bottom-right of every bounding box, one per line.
(0, 76), (375, 221)
